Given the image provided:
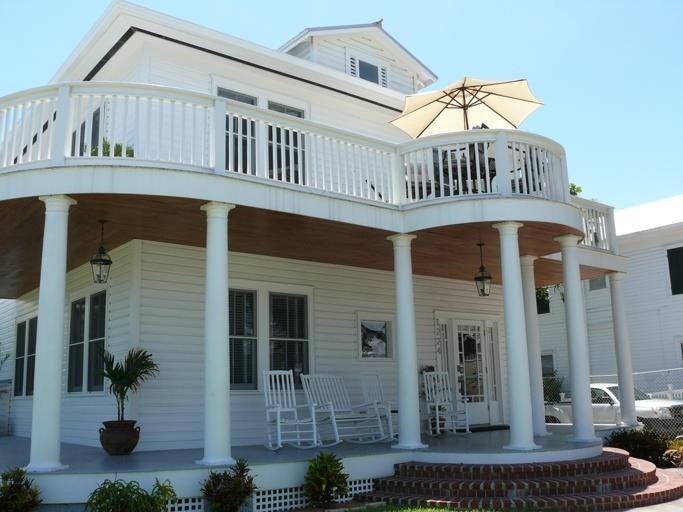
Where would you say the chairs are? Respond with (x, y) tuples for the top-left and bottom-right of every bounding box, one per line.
(423, 370), (472, 437)
(443, 123), (489, 195)
(510, 148), (541, 193)
(260, 369), (422, 451)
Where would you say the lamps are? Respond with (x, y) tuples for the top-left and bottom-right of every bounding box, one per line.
(90, 217), (112, 285)
(474, 241), (493, 297)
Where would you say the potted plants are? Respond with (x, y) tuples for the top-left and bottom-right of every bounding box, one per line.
(419, 365), (448, 434)
(94, 347), (161, 456)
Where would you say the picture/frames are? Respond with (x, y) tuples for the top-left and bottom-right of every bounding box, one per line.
(354, 310), (395, 362)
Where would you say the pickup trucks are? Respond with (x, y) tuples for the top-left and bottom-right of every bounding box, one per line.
(544, 383), (683, 431)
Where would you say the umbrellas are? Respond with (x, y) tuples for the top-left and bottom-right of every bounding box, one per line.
(385, 73), (547, 141)
